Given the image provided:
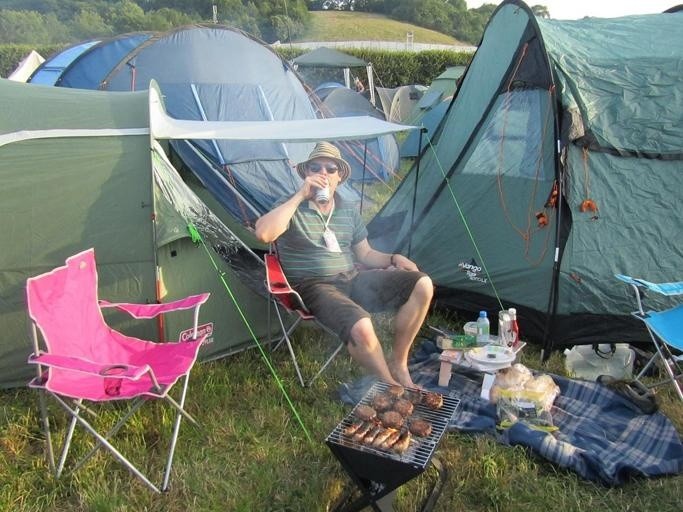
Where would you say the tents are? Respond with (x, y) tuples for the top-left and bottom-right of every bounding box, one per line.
(311, 79), (398, 180)
(407, 62), (465, 113)
(363, 0), (682, 356)
(358, 84), (428, 125)
(397, 98), (449, 158)
(0, 79), (298, 390)
(25, 24), (360, 225)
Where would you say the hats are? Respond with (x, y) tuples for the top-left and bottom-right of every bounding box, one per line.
(296, 141), (350, 188)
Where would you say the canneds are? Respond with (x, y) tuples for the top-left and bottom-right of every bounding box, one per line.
(314, 177), (330, 205)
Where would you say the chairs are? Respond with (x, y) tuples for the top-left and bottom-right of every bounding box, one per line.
(18, 248), (211, 494)
(264, 251), (345, 388)
(614, 272), (682, 399)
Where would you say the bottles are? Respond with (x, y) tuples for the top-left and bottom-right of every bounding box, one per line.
(476, 308), (519, 349)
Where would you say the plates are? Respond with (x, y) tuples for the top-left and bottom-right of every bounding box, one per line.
(468, 345), (515, 364)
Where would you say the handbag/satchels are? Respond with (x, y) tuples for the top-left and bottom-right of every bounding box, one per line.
(562, 341), (637, 383)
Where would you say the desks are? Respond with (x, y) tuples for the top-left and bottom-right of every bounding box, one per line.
(437, 334), (526, 402)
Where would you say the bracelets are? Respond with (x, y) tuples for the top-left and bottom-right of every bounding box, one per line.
(388, 251), (397, 268)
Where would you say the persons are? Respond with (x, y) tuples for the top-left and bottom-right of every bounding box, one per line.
(250, 139), (435, 392)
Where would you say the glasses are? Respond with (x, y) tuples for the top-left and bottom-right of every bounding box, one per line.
(307, 164), (339, 174)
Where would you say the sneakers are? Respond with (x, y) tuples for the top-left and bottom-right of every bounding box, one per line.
(596, 373), (662, 415)
